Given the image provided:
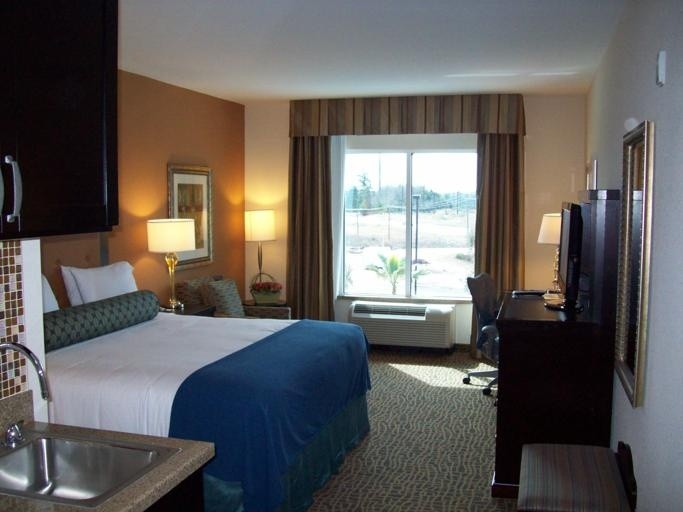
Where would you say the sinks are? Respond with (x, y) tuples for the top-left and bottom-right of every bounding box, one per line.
(0, 430), (182, 510)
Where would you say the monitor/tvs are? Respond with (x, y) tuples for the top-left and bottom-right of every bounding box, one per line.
(543, 201), (583, 314)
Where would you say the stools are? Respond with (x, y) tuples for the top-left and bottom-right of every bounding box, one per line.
(517, 441), (637, 512)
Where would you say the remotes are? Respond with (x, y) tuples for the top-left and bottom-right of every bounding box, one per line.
(511, 289), (545, 297)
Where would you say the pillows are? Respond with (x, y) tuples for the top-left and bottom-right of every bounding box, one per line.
(43, 290), (159, 354)
(201, 279), (245, 318)
(42, 261), (139, 314)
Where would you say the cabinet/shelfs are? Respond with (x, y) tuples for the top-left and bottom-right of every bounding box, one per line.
(0, 0), (119, 240)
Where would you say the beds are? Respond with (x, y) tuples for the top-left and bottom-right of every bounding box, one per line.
(45, 312), (372, 512)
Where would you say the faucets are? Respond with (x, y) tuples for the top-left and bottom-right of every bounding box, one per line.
(0, 342), (51, 402)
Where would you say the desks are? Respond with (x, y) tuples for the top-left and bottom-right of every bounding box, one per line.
(491, 290), (616, 498)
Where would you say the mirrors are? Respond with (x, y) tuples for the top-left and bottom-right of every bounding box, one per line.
(612, 119), (655, 409)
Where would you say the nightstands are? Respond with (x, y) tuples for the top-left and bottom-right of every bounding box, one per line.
(159, 303), (217, 316)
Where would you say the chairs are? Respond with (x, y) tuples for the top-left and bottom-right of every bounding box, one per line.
(174, 274), (291, 320)
(463, 273), (501, 395)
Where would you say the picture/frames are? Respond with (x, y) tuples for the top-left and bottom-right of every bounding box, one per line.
(167, 162), (214, 272)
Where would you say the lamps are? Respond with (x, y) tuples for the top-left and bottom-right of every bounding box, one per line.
(146, 218), (196, 311)
(537, 213), (561, 291)
(245, 209), (278, 282)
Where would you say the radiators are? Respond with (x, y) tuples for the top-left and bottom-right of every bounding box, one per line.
(349, 301), (456, 349)
